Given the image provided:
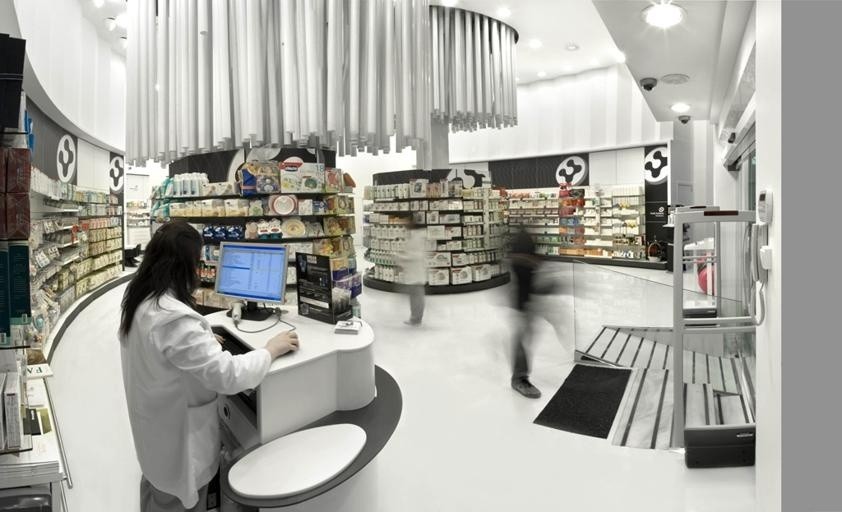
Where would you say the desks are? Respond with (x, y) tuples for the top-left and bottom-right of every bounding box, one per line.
(204, 304), (403, 511)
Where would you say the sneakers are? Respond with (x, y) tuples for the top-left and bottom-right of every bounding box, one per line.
(512, 378), (541, 398)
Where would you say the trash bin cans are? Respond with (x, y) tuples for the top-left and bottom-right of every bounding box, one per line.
(667, 243), (686, 271)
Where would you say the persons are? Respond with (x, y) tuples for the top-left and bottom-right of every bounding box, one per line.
(496, 217), (547, 401)
(119, 219), (301, 511)
(392, 210), (430, 327)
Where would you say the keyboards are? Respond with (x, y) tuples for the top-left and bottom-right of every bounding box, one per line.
(210, 326), (256, 357)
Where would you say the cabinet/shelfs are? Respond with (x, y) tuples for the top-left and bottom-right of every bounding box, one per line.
(503, 185), (641, 270)
(8, 147), (150, 363)
(153, 150), (365, 318)
(361, 169), (512, 294)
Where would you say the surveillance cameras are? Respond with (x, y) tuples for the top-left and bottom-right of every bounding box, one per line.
(679, 116), (690, 123)
(640, 77), (657, 91)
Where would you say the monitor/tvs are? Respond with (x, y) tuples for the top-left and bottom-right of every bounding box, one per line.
(215, 241), (290, 321)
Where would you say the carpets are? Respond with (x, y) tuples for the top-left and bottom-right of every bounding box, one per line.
(532, 364), (632, 439)
